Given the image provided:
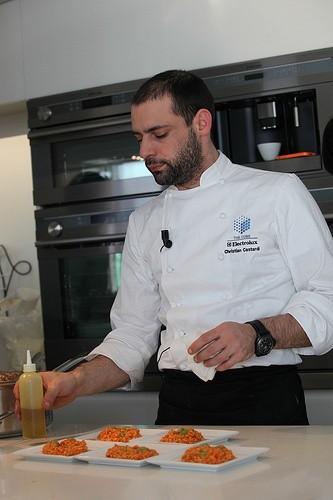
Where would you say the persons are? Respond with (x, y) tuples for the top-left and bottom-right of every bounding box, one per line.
(14, 70), (333, 425)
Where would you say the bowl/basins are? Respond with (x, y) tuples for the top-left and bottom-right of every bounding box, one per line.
(257, 142), (281, 161)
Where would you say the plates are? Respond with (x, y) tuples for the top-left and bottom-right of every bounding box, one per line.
(11, 426), (271, 472)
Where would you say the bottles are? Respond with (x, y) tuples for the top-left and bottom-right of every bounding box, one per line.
(18, 349), (48, 439)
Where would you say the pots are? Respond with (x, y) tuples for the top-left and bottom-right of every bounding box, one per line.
(0, 371), (26, 438)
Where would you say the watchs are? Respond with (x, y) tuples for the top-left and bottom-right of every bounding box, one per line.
(245, 320), (276, 357)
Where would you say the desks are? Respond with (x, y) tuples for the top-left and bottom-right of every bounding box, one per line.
(0, 425), (333, 500)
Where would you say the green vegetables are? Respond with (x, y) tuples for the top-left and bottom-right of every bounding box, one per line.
(51, 428), (209, 457)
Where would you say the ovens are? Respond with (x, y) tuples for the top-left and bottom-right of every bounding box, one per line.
(25, 44), (333, 393)
